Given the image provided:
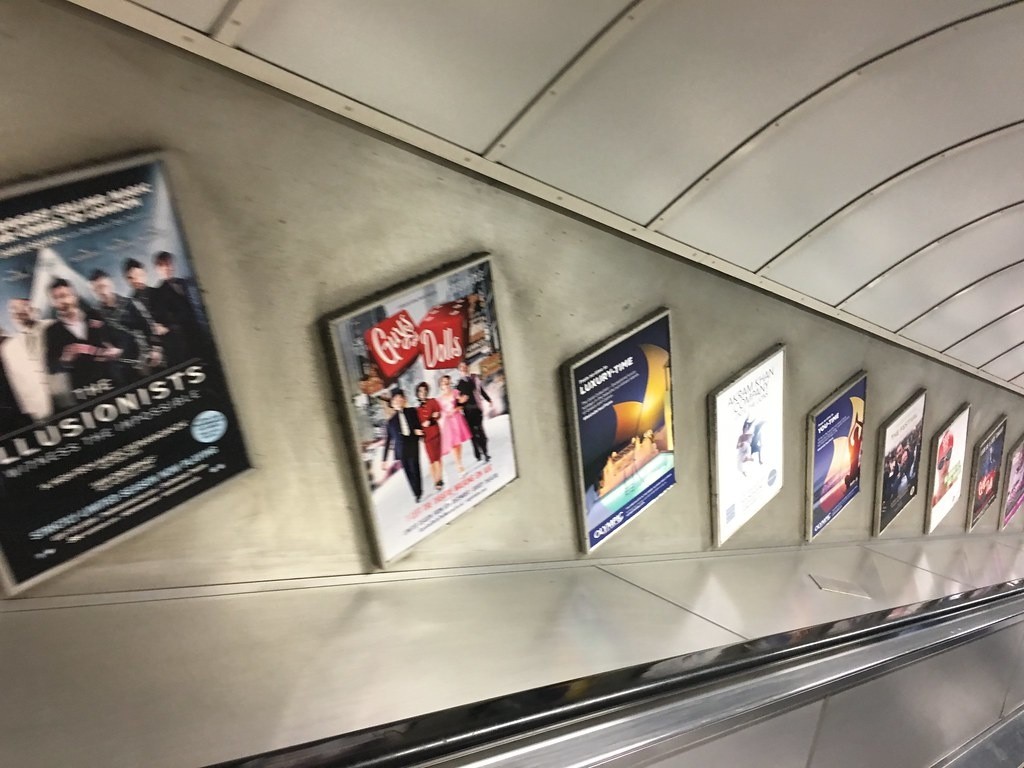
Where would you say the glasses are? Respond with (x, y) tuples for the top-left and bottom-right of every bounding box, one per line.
(938, 448), (952, 470)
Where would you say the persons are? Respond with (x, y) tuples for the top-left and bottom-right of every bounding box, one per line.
(0, 251), (202, 436)
(381, 361), (493, 503)
(883, 426), (923, 509)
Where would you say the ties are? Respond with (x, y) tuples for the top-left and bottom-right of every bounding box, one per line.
(466, 377), (471, 381)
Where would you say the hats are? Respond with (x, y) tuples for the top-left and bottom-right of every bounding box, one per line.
(392, 388), (403, 396)
(934, 434), (954, 462)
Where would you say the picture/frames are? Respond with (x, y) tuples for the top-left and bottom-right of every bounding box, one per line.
(802, 369), (870, 544)
(997, 432), (1024, 532)
(923, 402), (972, 537)
(705, 341), (789, 550)
(0, 141), (257, 599)
(561, 305), (680, 557)
(319, 251), (521, 572)
(872, 388), (928, 539)
(965, 414), (1009, 535)
